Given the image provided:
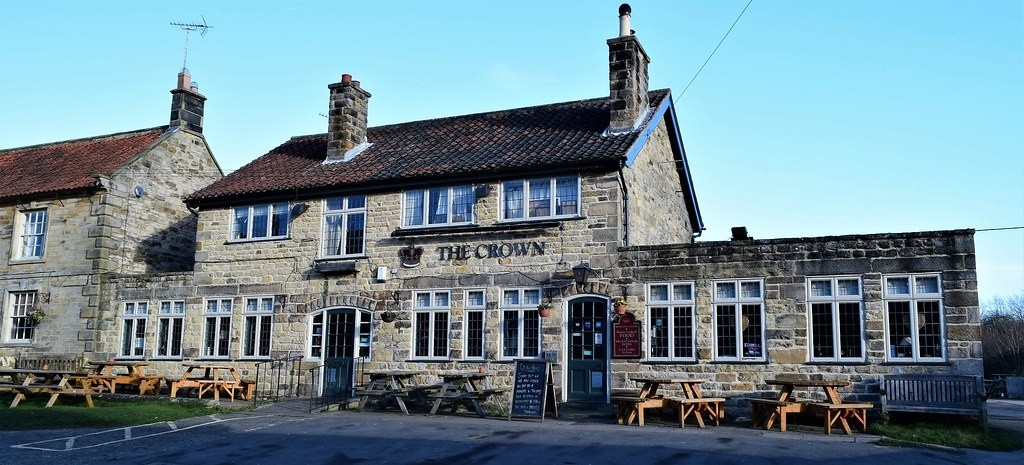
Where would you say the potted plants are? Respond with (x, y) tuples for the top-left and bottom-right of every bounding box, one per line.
(538, 305), (552, 317)
(381, 311), (394, 323)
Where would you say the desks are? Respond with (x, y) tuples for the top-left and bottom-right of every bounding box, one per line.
(0, 369), (96, 408)
(765, 380), (850, 434)
(429, 372), (507, 418)
(358, 370), (428, 415)
(88, 361), (151, 395)
(628, 378), (710, 428)
(167, 362), (255, 399)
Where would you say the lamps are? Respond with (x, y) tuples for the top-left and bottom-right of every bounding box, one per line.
(572, 261), (589, 284)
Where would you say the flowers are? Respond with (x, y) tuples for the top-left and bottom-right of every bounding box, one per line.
(615, 300), (626, 307)
(27, 307), (44, 322)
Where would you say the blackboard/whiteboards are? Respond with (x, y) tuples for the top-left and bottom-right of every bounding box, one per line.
(509, 356), (550, 418)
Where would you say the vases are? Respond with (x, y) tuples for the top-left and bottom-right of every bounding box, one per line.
(617, 306), (625, 314)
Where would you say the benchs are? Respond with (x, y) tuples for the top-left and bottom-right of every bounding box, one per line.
(813, 403), (873, 435)
(76, 375), (115, 395)
(195, 380), (234, 402)
(611, 397), (662, 427)
(18, 351), (88, 384)
(429, 393), (488, 419)
(356, 390), (408, 415)
(748, 397), (786, 433)
(677, 398), (724, 427)
(883, 374), (989, 436)
